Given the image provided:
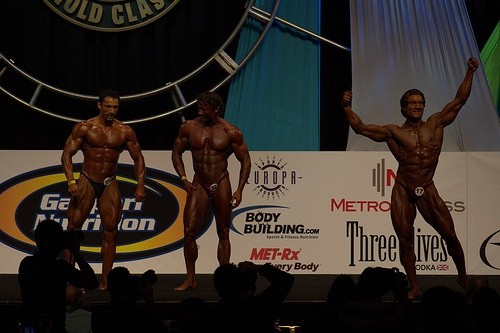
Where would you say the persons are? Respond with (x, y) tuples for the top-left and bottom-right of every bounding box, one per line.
(340, 56), (479, 300)
(17, 218), (500, 333)
(171, 91), (251, 291)
(61, 89), (146, 291)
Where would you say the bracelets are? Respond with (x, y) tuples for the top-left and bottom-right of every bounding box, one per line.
(180, 175), (187, 182)
(341, 100), (349, 107)
(67, 179), (76, 186)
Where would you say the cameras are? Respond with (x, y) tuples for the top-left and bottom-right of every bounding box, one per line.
(64, 230), (84, 245)
(132, 270), (156, 291)
